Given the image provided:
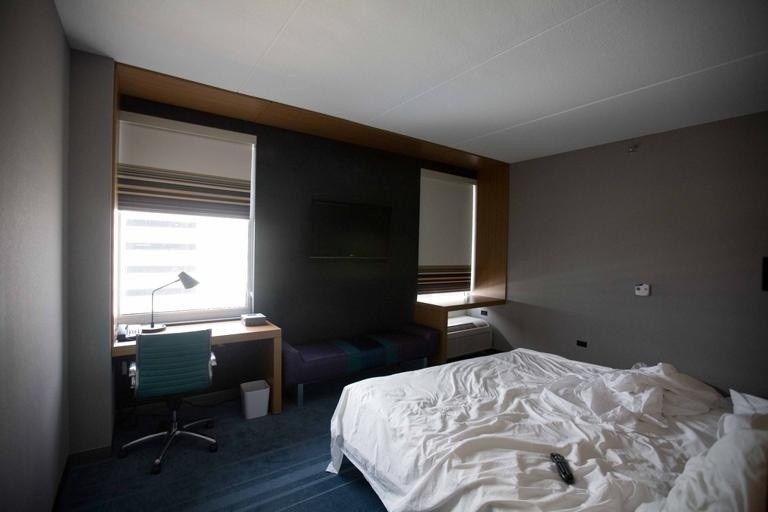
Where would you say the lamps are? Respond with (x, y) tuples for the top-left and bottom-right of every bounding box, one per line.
(141, 271), (199, 333)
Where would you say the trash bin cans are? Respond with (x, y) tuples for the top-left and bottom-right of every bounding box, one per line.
(241, 380), (269, 419)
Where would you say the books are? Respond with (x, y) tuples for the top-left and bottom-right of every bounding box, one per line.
(241, 312), (267, 327)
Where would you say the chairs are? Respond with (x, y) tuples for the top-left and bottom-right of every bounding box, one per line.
(121, 329), (218, 474)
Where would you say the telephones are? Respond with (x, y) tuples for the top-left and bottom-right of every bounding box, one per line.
(116, 323), (142, 342)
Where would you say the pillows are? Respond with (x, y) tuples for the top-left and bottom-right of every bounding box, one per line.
(717, 389), (768, 439)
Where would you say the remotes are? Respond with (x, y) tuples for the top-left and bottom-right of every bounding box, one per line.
(550, 451), (575, 484)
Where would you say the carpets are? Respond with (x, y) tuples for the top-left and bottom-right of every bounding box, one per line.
(54, 362), (427, 512)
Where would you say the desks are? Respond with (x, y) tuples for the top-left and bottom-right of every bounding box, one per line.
(112, 320), (282, 415)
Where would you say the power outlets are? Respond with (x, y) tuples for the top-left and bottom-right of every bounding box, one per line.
(634, 284), (650, 296)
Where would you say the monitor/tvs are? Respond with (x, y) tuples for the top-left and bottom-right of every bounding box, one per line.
(306, 199), (395, 264)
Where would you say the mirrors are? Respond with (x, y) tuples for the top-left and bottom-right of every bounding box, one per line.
(417, 169), (476, 298)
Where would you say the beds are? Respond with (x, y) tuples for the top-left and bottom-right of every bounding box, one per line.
(326, 348), (767, 512)
(282, 322), (444, 407)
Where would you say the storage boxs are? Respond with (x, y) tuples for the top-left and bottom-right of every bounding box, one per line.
(446, 314), (493, 356)
(382, 330), (414, 364)
(345, 336), (387, 367)
